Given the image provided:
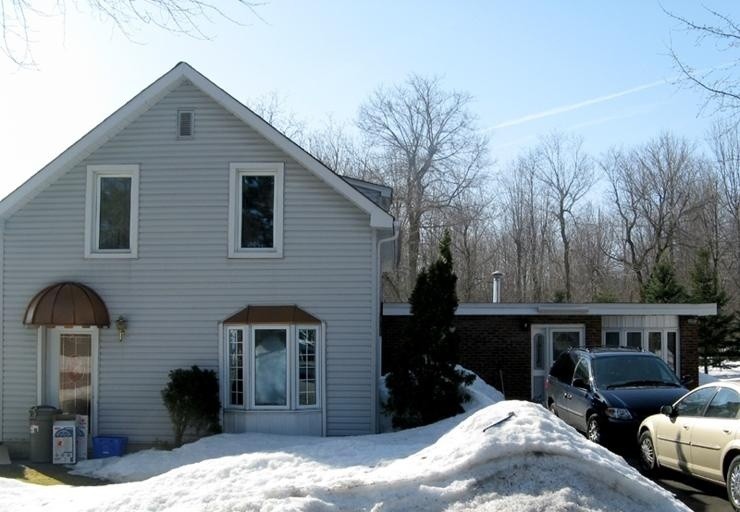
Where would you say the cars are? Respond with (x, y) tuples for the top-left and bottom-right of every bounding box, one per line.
(635, 377), (740, 511)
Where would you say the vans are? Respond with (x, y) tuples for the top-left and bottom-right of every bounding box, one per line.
(542, 345), (692, 449)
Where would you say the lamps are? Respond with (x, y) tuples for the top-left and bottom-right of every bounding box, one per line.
(114, 314), (127, 342)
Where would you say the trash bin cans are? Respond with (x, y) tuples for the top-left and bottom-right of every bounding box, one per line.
(92, 436), (129, 459)
(27, 405), (63, 464)
